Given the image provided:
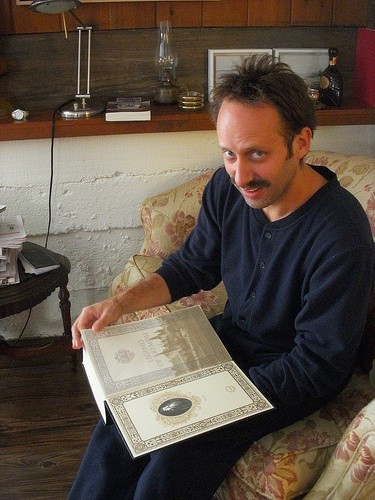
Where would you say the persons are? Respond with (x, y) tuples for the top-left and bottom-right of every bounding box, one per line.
(67, 53), (375, 500)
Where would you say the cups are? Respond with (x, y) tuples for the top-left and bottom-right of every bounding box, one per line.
(309, 89), (320, 109)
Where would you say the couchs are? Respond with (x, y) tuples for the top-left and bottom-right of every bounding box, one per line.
(109, 150), (375, 500)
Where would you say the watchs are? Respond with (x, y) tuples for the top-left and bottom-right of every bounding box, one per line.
(11, 109), (29, 120)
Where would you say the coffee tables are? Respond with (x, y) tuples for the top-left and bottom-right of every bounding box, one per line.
(0, 241), (76, 371)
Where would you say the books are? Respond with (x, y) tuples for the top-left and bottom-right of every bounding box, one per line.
(79, 304), (274, 459)
(105, 100), (151, 122)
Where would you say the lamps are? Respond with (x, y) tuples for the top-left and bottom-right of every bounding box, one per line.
(28, 0), (106, 119)
(154, 20), (181, 104)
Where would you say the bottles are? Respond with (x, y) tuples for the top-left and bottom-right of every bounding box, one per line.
(319, 47), (344, 106)
